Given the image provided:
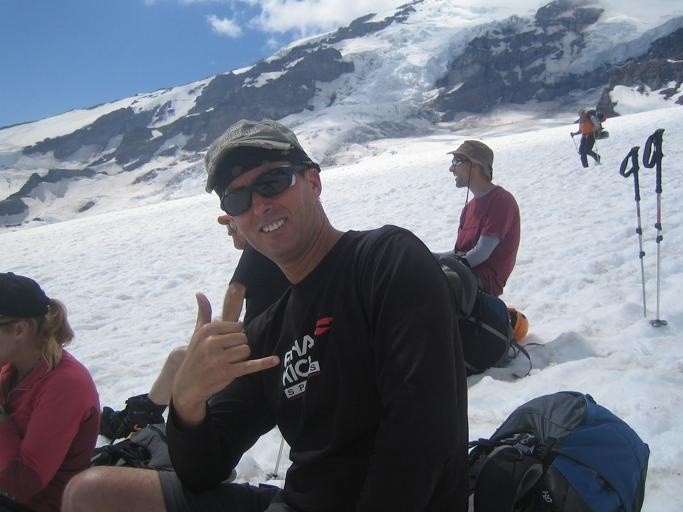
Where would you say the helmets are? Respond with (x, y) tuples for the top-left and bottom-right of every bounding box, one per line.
(505, 304), (529, 344)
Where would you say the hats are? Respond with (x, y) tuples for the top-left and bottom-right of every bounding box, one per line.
(202, 117), (304, 195)
(446, 137), (493, 181)
(0, 272), (49, 319)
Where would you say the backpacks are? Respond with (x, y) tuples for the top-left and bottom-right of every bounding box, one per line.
(465, 389), (649, 511)
(446, 259), (510, 381)
(587, 109), (607, 139)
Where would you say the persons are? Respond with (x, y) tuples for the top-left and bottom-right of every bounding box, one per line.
(571, 109), (603, 168)
(61, 116), (469, 512)
(428, 139), (521, 299)
(0, 271), (100, 512)
(90, 214), (287, 469)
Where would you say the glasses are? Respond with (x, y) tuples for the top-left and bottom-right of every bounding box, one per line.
(450, 157), (468, 167)
(218, 164), (306, 217)
